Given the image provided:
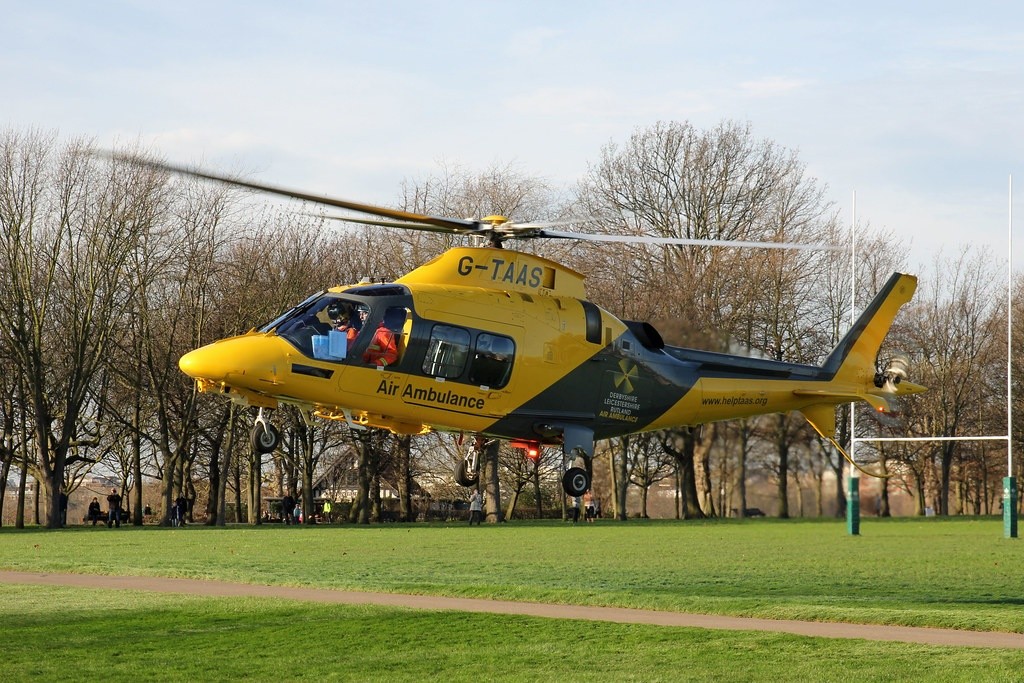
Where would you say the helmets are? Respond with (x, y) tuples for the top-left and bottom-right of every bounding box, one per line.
(331, 311), (351, 324)
(354, 300), (369, 312)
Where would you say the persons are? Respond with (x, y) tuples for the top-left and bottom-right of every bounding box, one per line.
(171, 491), (189, 527)
(572, 496), (582, 523)
(144, 503), (152, 515)
(106, 488), (121, 528)
(323, 499), (331, 524)
(330, 314), (357, 352)
(357, 306), (399, 368)
(283, 491), (296, 525)
(584, 488), (602, 522)
(58, 488), (67, 525)
(89, 497), (100, 526)
(469, 489), (483, 526)
(294, 504), (302, 524)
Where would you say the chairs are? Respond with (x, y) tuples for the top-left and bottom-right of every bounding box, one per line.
(474, 338), (509, 390)
(344, 314), (362, 332)
(384, 308), (407, 349)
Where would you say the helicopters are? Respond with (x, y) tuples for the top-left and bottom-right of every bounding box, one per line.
(70, 147), (928, 498)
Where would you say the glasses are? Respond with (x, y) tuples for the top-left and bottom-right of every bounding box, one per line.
(337, 321), (344, 326)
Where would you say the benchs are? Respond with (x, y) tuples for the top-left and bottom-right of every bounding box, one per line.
(733, 508), (766, 518)
(83, 514), (127, 526)
